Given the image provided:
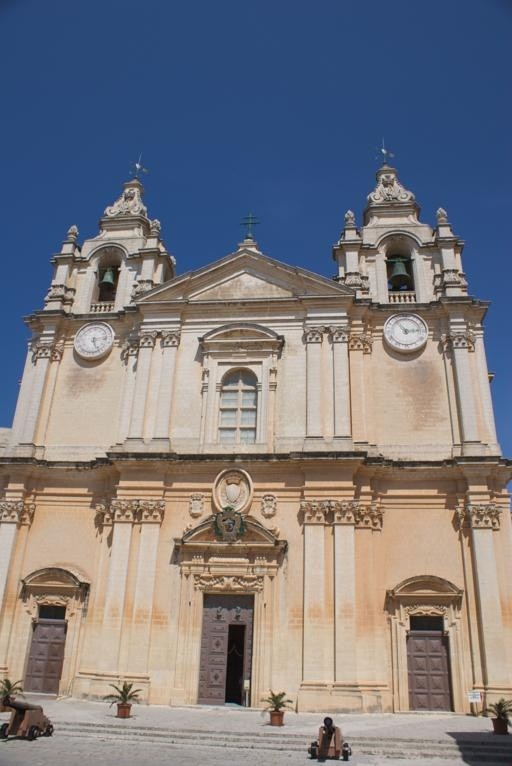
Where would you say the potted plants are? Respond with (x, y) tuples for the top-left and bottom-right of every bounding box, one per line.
(260, 689), (296, 726)
(102, 679), (143, 718)
(477, 698), (512, 736)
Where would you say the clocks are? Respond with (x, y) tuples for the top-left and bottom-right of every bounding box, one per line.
(74, 320), (116, 361)
(383, 310), (429, 355)
